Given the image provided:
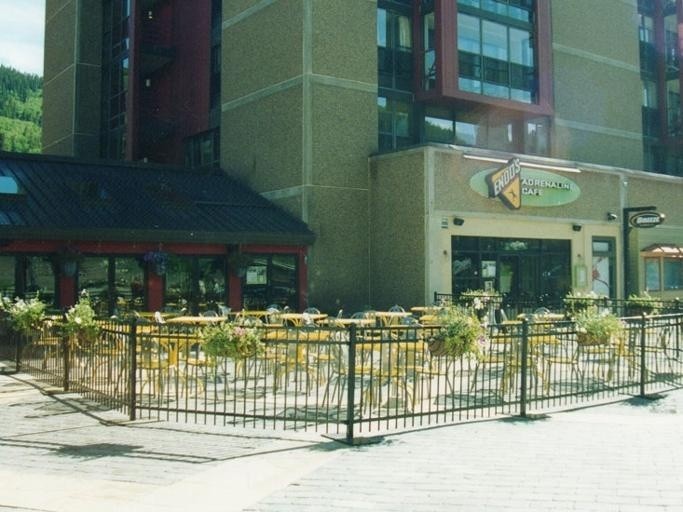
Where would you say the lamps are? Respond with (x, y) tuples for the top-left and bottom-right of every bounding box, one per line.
(572, 223), (582, 231)
(454, 218), (464, 225)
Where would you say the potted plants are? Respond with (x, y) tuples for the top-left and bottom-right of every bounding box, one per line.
(226, 251), (256, 278)
(142, 250), (181, 275)
(54, 250), (85, 277)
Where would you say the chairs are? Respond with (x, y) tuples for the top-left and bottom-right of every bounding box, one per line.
(0, 290), (674, 418)
